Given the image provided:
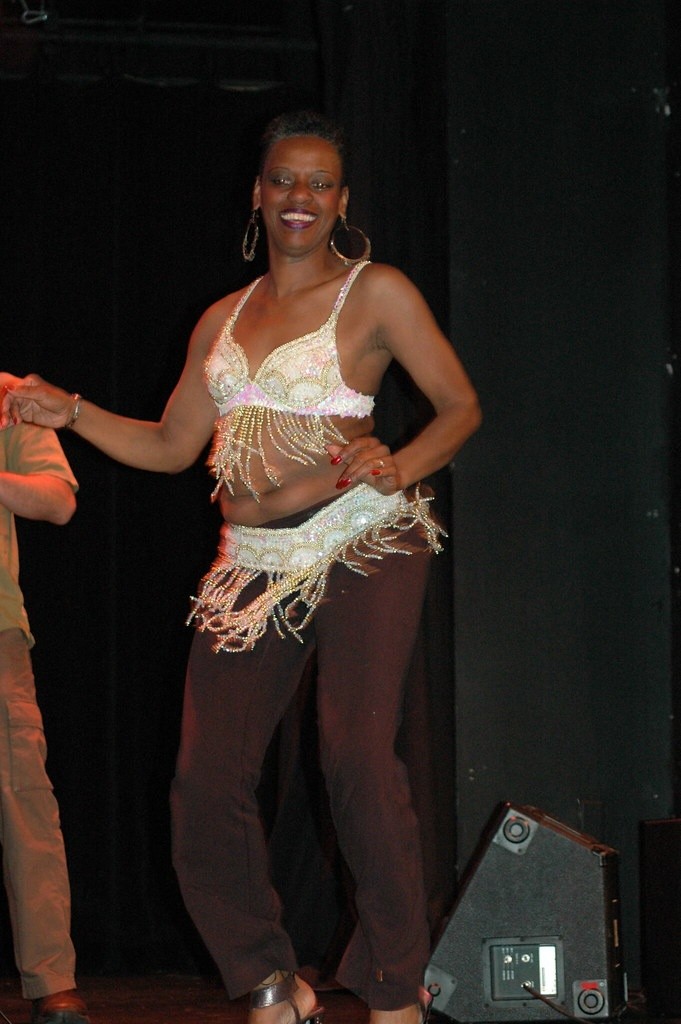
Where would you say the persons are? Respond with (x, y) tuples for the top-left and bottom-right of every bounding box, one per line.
(0, 369), (86, 1024)
(0, 111), (484, 1024)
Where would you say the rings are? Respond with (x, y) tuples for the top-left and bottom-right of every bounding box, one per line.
(378, 458), (386, 468)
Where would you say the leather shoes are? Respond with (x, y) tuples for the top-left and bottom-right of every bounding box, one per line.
(32, 992), (91, 1023)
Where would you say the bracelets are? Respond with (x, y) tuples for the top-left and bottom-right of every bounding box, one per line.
(64, 393), (81, 427)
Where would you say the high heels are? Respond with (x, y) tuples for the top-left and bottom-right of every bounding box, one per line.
(247, 980), (432, 1024)
